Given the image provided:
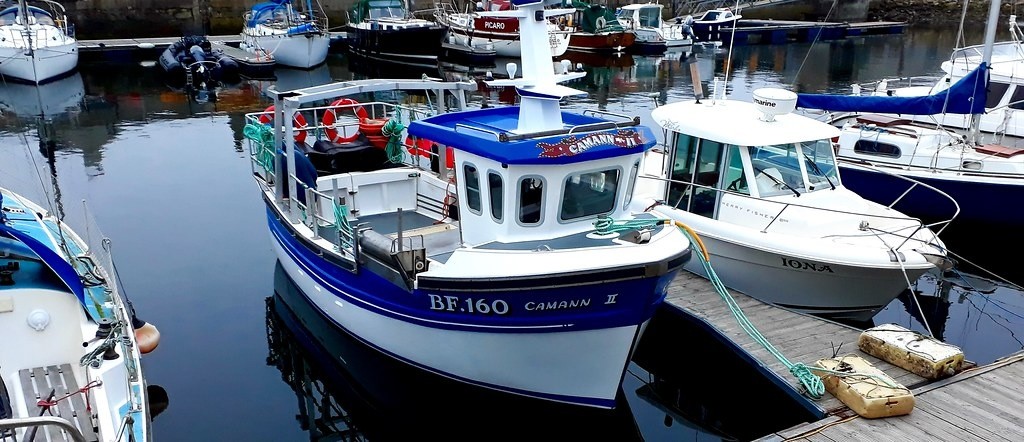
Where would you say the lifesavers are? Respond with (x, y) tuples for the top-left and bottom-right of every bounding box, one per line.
(323, 96), (369, 144)
(259, 103), (308, 143)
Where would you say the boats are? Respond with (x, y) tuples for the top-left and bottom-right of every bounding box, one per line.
(337, 0), (1024, 236)
(565, 0), (961, 323)
(243, 0), (692, 412)
(210, 35), (279, 81)
(158, 32), (242, 99)
(0, 189), (154, 442)
(240, 0), (331, 70)
(0, 69), (86, 220)
(240, 61), (334, 100)
(0, 0), (79, 85)
